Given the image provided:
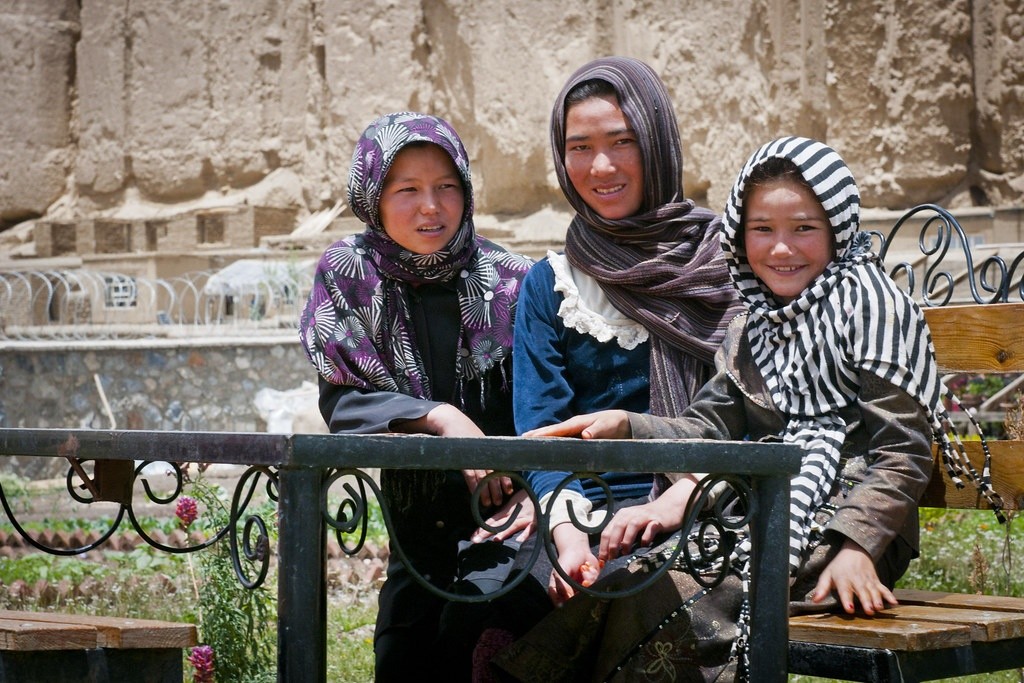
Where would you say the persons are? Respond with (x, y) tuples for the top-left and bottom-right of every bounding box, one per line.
(462, 53), (748, 683)
(297, 111), (540, 683)
(487, 135), (937, 683)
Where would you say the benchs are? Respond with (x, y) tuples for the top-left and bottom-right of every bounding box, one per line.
(792, 302), (1024, 683)
(0, 609), (201, 683)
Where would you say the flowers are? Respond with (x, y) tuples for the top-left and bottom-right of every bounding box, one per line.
(176, 476), (278, 683)
